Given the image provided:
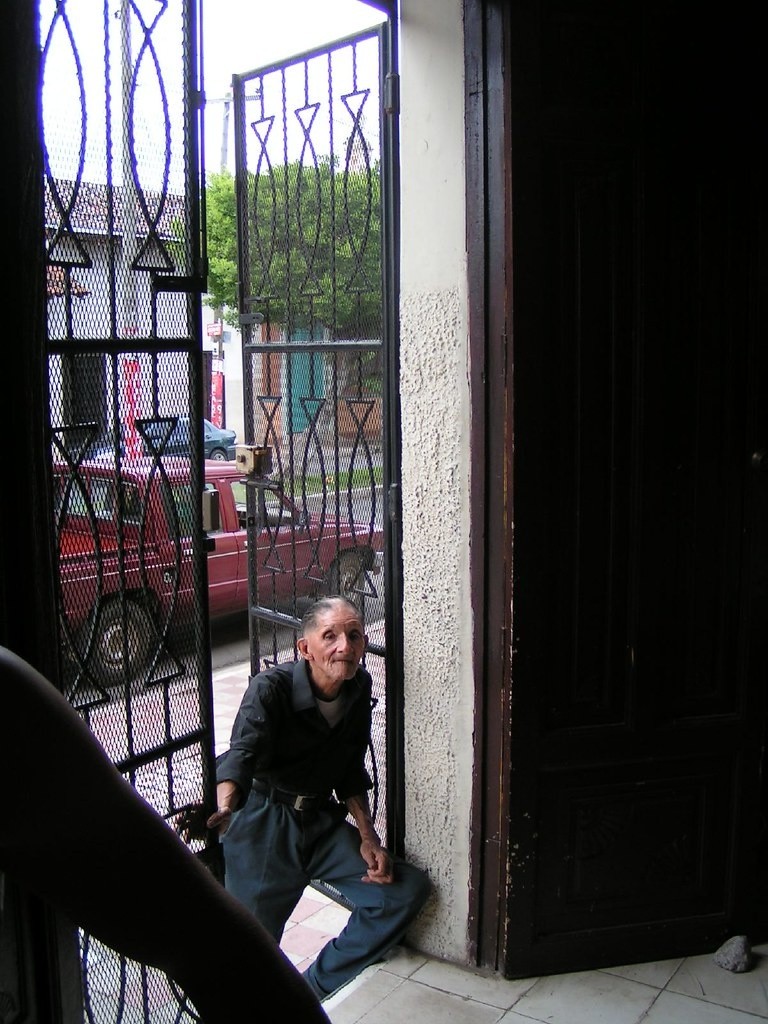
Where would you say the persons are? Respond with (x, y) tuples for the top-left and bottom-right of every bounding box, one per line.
(0, 644), (332, 1024)
(173, 593), (434, 1003)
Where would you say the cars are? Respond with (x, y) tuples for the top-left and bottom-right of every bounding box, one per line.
(70, 417), (238, 464)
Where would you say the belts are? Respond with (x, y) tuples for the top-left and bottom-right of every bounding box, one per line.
(252, 778), (327, 812)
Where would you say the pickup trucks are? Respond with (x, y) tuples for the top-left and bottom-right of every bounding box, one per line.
(54, 457), (383, 689)
